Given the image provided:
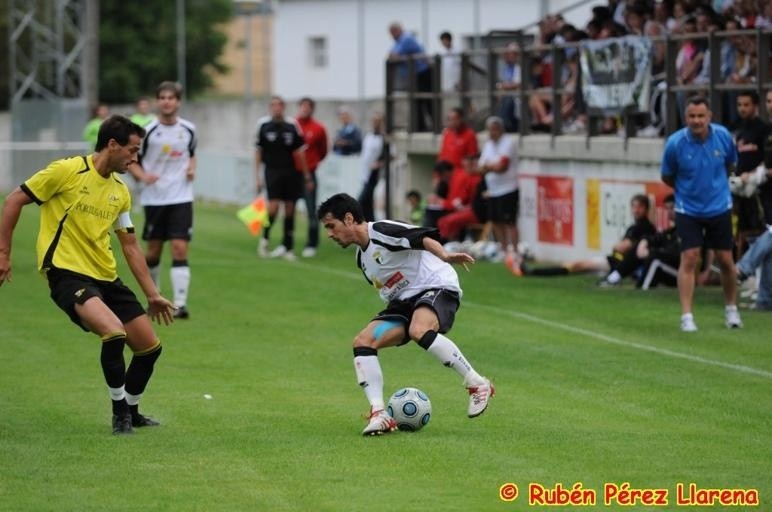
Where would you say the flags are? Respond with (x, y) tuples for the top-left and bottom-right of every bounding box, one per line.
(237, 194), (270, 234)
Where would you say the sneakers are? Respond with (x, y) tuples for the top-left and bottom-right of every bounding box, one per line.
(724, 310), (742, 328)
(468, 376), (495, 417)
(596, 278), (621, 289)
(113, 407), (132, 435)
(257, 237), (318, 259)
(173, 305), (187, 318)
(133, 415), (159, 427)
(363, 408), (399, 436)
(680, 317), (698, 332)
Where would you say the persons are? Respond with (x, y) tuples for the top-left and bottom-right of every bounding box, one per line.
(334, 106), (361, 155)
(318, 193), (494, 436)
(283, 97), (327, 258)
(84, 102), (112, 142)
(351, 112), (385, 221)
(131, 97), (160, 128)
(253, 95), (315, 260)
(0, 116), (178, 432)
(127, 81), (197, 317)
(378, 1), (771, 331)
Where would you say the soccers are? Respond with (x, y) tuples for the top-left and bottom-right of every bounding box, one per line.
(386, 387), (431, 430)
